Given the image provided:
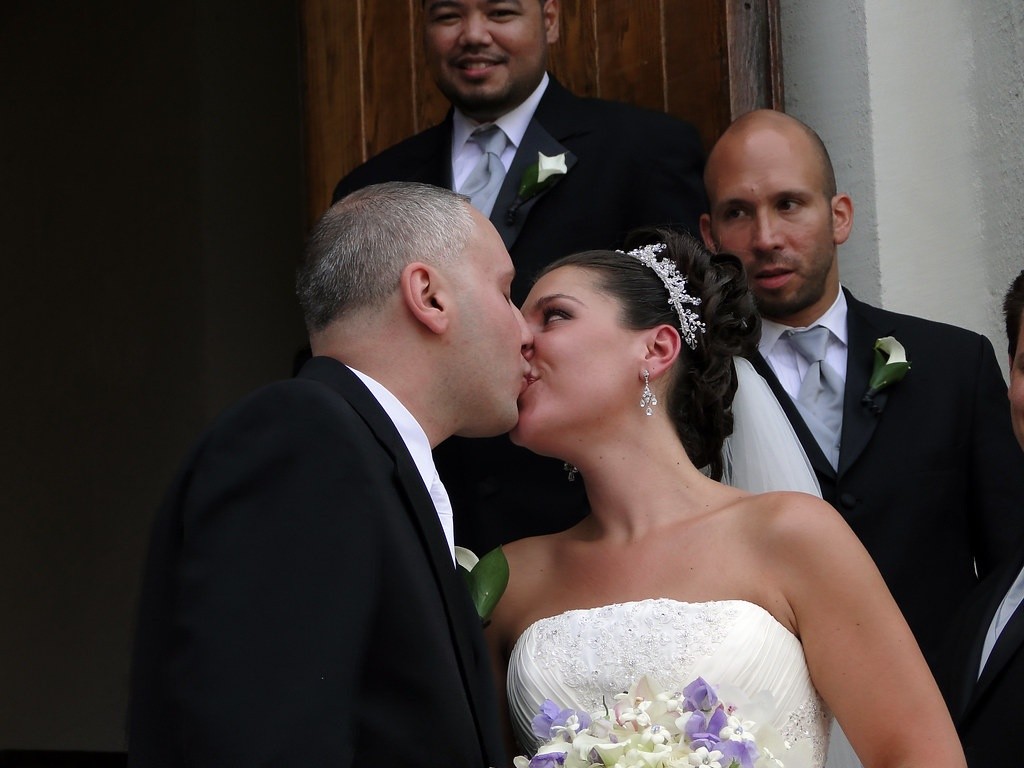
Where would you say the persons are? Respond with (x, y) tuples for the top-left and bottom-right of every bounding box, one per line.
(954, 263), (1024, 768)
(326, 0), (712, 581)
(463, 222), (969, 767)
(122, 180), (535, 768)
(694, 106), (1024, 751)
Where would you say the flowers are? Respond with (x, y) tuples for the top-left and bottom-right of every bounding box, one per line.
(505, 151), (568, 225)
(514, 673), (760, 768)
(454, 544), (510, 628)
(862, 337), (912, 416)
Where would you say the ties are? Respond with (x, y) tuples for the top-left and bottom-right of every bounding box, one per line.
(458, 127), (509, 219)
(430, 469), (456, 572)
(995, 567), (1024, 640)
(782, 325), (845, 433)
